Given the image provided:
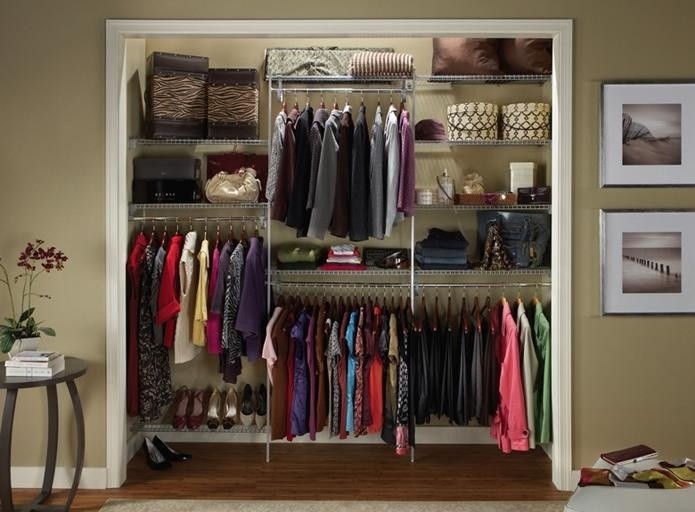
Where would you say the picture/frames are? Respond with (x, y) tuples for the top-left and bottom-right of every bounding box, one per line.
(598, 81), (695, 188)
(597, 208), (695, 317)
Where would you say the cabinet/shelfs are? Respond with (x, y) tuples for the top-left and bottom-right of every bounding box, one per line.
(104, 19), (572, 492)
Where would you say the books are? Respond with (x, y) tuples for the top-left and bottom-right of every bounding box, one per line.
(5, 350), (65, 376)
(601, 444), (657, 466)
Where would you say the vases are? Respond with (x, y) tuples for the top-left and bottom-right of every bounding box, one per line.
(8, 334), (41, 359)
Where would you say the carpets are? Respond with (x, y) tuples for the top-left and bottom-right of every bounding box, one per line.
(97, 495), (566, 511)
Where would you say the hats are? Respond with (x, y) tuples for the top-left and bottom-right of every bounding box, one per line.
(414, 119), (449, 140)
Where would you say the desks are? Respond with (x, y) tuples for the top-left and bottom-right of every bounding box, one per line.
(0, 356), (89, 511)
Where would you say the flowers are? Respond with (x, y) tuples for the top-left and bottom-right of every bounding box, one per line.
(0, 238), (68, 353)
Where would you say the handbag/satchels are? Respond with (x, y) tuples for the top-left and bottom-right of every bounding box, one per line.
(476, 210), (550, 268)
(480, 222), (511, 270)
(204, 168), (262, 205)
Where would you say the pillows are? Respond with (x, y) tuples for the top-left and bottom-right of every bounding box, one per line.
(431, 36), (497, 75)
(501, 37), (550, 75)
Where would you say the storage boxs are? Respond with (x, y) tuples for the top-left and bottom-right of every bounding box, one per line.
(133, 158), (201, 181)
(131, 179), (203, 203)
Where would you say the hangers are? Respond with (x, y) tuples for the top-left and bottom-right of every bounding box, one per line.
(135, 217), (262, 253)
(275, 88), (410, 123)
(269, 278), (543, 334)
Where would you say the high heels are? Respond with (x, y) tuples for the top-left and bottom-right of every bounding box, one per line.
(142, 383), (267, 471)
